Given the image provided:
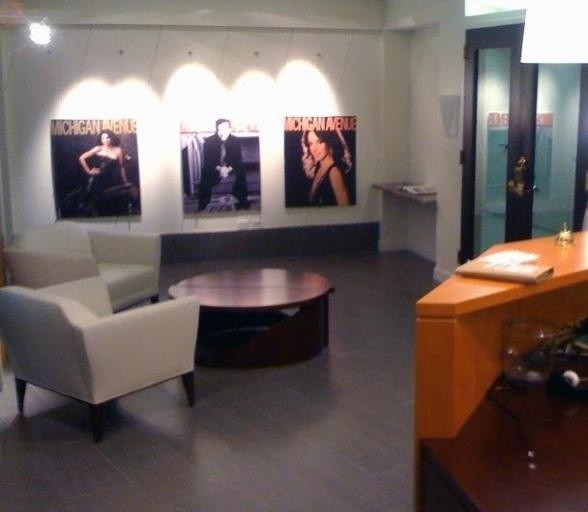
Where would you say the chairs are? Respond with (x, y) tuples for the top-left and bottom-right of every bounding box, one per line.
(0, 275), (202, 445)
(2, 221), (163, 314)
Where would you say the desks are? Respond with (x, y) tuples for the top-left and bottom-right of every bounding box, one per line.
(169, 268), (336, 369)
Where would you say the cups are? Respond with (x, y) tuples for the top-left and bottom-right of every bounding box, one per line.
(501, 316), (559, 389)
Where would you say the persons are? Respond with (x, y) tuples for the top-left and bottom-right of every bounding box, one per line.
(196, 120), (251, 211)
(301, 127), (353, 207)
(79, 130), (129, 215)
